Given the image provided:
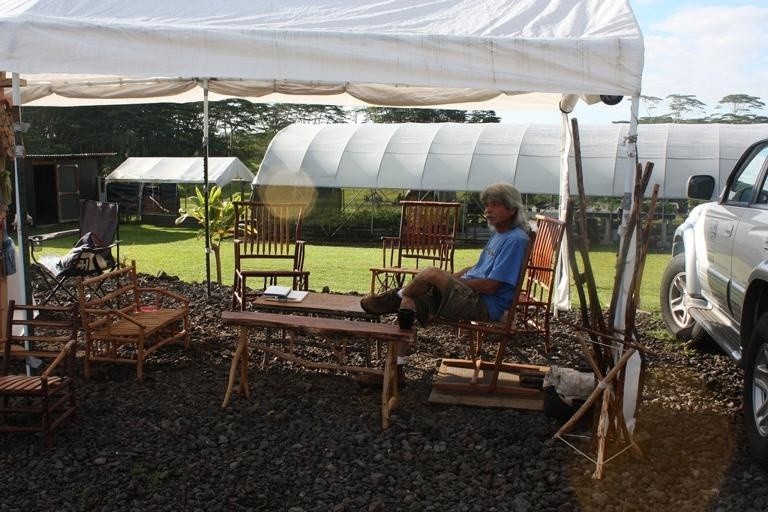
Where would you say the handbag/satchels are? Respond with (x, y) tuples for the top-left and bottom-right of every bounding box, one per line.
(56, 232), (113, 271)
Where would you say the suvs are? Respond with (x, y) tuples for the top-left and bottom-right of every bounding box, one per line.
(655, 135), (767, 438)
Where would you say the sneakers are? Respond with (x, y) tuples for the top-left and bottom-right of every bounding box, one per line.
(358, 369), (405, 387)
(360, 287), (401, 314)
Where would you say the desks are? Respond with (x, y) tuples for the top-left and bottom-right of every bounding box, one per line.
(253, 289), (381, 378)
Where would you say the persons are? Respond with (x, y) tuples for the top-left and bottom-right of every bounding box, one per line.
(361, 182), (533, 332)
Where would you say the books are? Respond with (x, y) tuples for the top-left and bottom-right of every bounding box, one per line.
(263, 285), (310, 303)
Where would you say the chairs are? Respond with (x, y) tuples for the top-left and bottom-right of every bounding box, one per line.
(227, 199), (310, 310)
(431, 213), (566, 396)
(26, 198), (120, 318)
(368, 200), (462, 298)
(0, 299), (80, 447)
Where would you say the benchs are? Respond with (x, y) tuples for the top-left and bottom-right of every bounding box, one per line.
(219, 311), (418, 430)
(76, 261), (195, 377)
